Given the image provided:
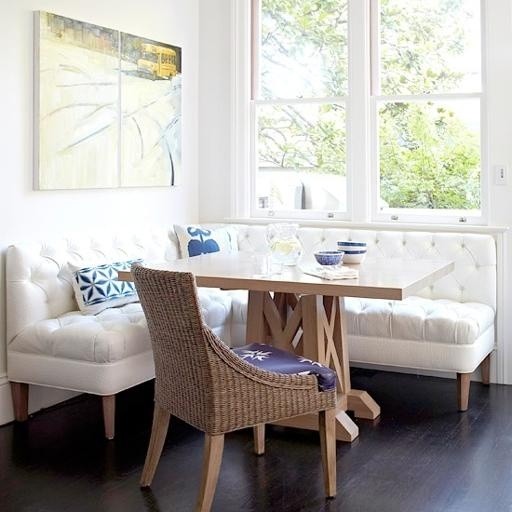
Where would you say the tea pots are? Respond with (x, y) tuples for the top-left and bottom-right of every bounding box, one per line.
(265, 222), (305, 267)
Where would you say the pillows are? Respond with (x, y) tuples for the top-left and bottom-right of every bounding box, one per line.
(68, 259), (144, 314)
(174, 224), (240, 259)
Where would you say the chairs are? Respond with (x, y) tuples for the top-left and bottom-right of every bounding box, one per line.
(128, 264), (338, 512)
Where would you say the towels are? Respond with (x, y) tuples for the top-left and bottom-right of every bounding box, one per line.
(303, 264), (359, 281)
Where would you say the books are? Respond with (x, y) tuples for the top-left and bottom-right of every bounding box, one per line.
(306, 264), (361, 282)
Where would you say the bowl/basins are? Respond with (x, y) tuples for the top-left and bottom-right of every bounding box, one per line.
(337, 241), (367, 249)
(338, 249), (367, 264)
(314, 250), (344, 265)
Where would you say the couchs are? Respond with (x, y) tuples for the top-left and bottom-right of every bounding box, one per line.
(7, 223), (498, 439)
